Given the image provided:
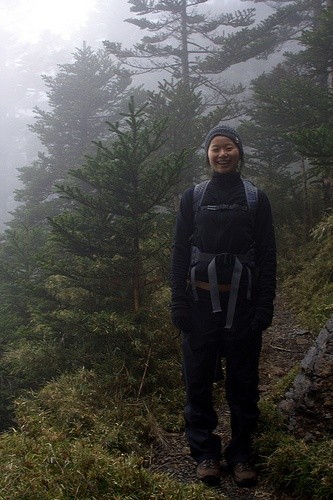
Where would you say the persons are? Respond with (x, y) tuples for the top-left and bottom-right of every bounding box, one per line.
(169, 122), (278, 487)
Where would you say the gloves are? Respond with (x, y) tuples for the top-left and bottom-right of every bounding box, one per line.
(170, 305), (193, 335)
(251, 305), (273, 332)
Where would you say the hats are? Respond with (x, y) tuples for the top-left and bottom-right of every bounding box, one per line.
(204, 125), (242, 160)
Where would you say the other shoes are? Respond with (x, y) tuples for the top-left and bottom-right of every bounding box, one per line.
(232, 462), (257, 484)
(196, 460), (220, 480)
